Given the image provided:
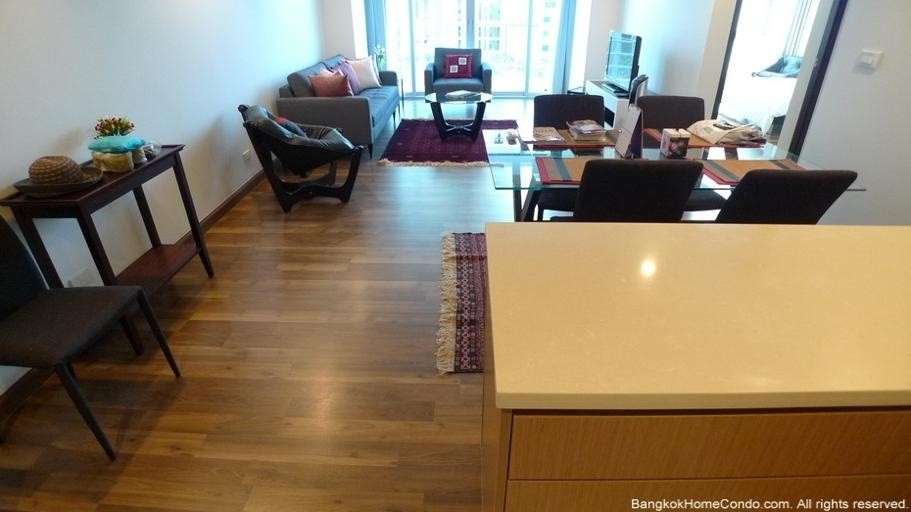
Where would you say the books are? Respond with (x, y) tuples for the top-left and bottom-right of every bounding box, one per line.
(517, 126), (565, 142)
(569, 119), (607, 141)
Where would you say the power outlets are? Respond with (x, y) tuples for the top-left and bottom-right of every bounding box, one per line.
(242, 148), (252, 161)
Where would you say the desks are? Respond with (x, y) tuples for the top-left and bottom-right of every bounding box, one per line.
(424, 90), (493, 141)
(481, 128), (828, 223)
(1, 144), (214, 355)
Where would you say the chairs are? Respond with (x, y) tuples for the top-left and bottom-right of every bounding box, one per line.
(752, 55), (802, 77)
(534, 94), (604, 221)
(637, 95), (728, 211)
(0, 217), (181, 460)
(551, 158), (703, 222)
(237, 104), (365, 213)
(714, 169), (858, 224)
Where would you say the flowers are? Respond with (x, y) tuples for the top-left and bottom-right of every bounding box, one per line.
(92, 116), (135, 139)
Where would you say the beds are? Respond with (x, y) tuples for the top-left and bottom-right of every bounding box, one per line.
(720, 77), (797, 132)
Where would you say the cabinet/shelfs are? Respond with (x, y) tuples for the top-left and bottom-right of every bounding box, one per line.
(482, 221), (911, 512)
(585, 80), (654, 130)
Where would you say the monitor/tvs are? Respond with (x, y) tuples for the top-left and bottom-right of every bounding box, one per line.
(603, 30), (642, 94)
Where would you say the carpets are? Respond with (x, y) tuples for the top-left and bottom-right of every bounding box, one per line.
(433, 232), (483, 376)
(377, 118), (518, 169)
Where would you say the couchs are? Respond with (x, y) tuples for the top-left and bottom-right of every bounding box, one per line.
(276, 54), (400, 159)
(424, 48), (492, 103)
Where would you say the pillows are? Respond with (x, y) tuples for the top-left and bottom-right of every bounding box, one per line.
(308, 55), (382, 95)
(444, 53), (472, 78)
(274, 116), (308, 138)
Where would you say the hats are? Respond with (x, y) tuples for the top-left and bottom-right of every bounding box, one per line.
(13, 155), (104, 196)
